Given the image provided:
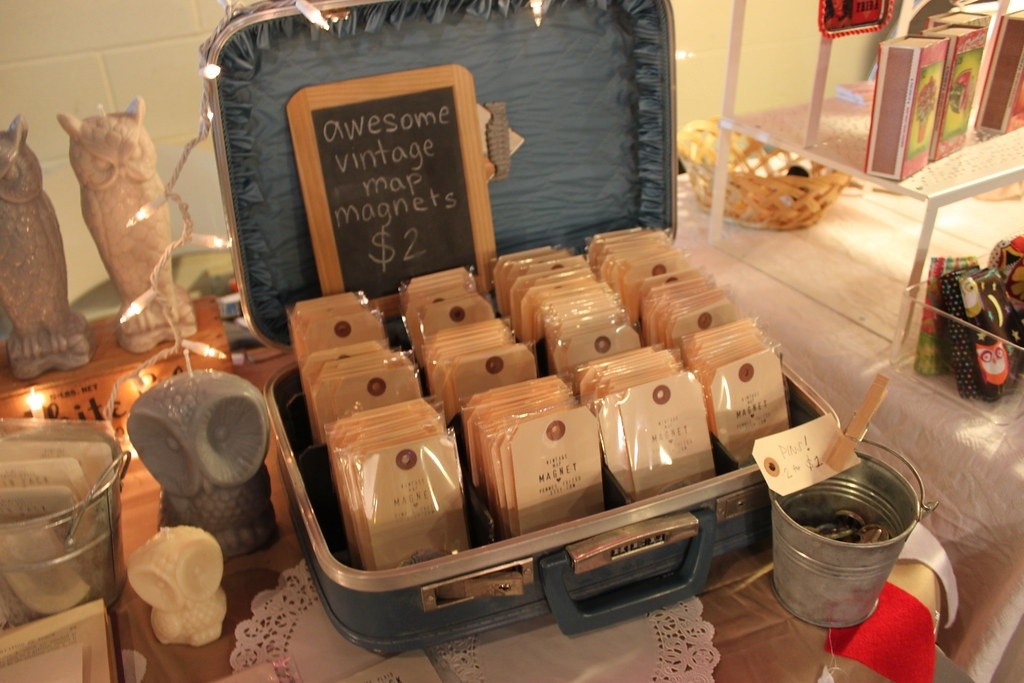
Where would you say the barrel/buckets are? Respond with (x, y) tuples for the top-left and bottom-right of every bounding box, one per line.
(769, 432), (939, 629)
(1, 421), (129, 619)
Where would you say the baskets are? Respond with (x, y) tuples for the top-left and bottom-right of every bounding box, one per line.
(677, 116), (848, 231)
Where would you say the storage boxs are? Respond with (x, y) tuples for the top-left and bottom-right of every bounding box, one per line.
(0, 297), (230, 438)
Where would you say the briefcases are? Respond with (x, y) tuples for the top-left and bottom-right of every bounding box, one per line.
(204, 0), (841, 652)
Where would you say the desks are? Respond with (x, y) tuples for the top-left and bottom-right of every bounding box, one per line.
(0, 93), (1024, 683)
